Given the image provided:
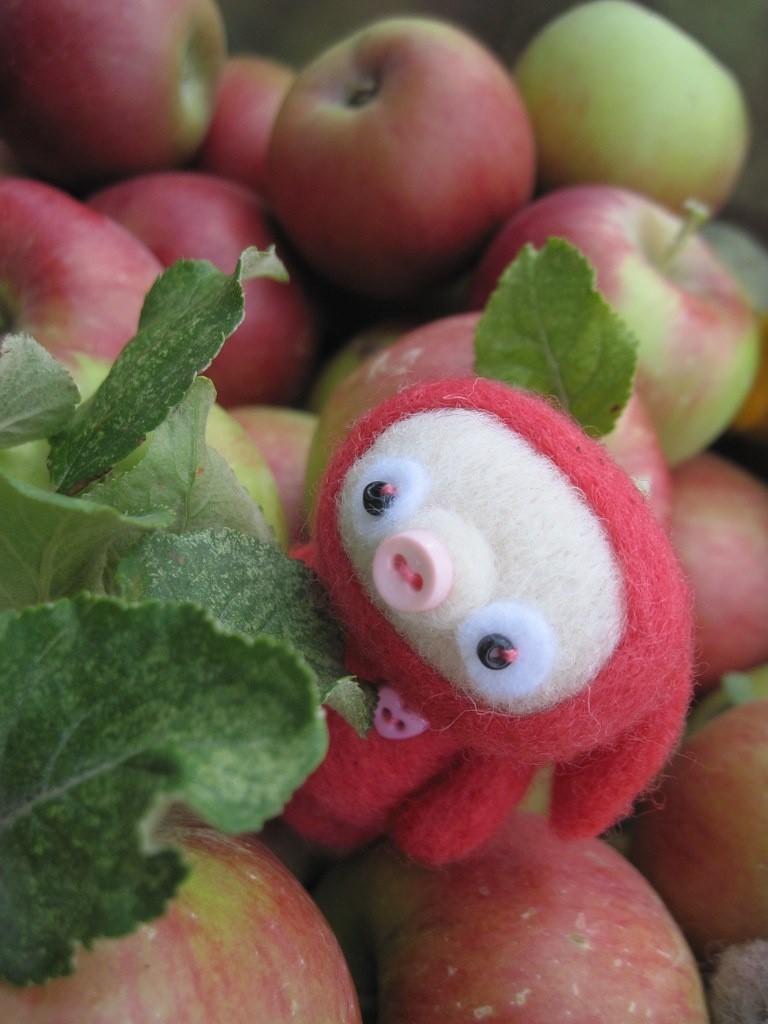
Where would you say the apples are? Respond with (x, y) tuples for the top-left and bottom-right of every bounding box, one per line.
(4, 0), (768, 1022)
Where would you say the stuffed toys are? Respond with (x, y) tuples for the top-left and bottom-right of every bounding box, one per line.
(277, 379), (698, 865)
